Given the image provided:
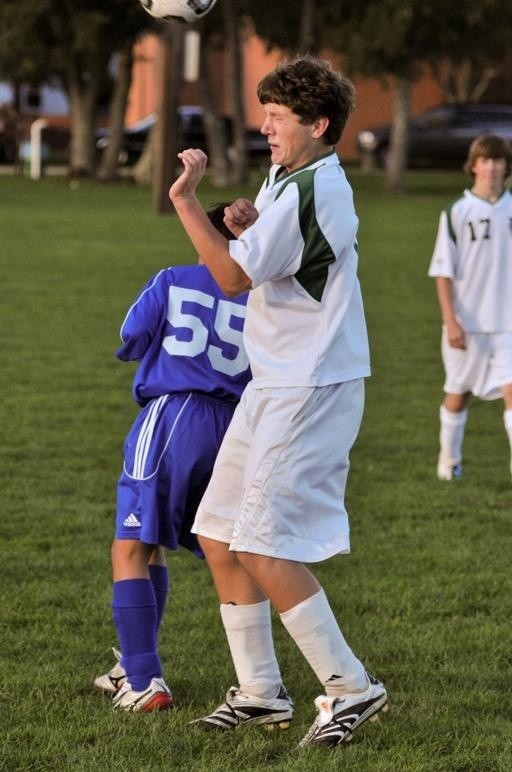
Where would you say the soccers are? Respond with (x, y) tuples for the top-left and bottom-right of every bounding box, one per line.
(139, 1), (215, 25)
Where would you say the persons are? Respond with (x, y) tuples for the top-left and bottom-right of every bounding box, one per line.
(95, 207), (253, 715)
(168, 52), (389, 754)
(427, 134), (512, 483)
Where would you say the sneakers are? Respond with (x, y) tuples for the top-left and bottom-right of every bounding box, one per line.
(93, 647), (172, 713)
(436, 457), (463, 481)
(186, 671), (388, 749)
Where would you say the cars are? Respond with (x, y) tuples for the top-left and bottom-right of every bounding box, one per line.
(95, 107), (271, 166)
(358, 103), (512, 167)
